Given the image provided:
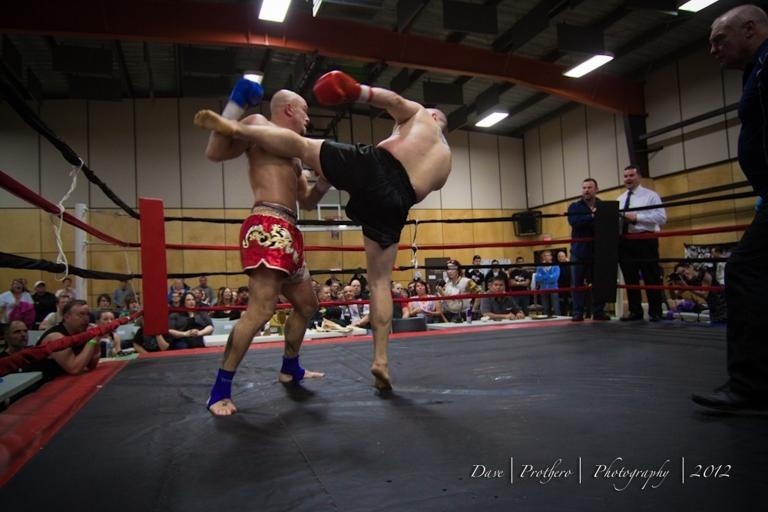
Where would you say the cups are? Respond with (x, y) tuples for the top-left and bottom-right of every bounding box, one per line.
(504, 304), (513, 314)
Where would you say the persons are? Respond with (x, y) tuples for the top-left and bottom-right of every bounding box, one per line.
(615, 164), (668, 323)
(205, 69), (326, 416)
(567, 178), (610, 321)
(0, 246), (727, 411)
(689, 4), (764, 412)
(194, 70), (452, 390)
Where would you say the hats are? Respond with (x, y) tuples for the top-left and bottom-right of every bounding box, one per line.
(34, 280), (46, 290)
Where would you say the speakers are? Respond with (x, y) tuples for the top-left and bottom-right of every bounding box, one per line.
(533, 248), (567, 266)
(425, 257), (450, 284)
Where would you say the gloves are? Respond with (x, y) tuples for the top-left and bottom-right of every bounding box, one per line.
(312, 69), (373, 106)
(222, 77), (264, 120)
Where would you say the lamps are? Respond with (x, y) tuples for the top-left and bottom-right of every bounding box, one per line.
(474, 106), (510, 129)
(258, 0), (292, 23)
(678, 0), (721, 13)
(562, 51), (615, 79)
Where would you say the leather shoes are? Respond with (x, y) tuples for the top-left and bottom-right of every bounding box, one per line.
(649, 315), (661, 322)
(620, 311), (644, 321)
(593, 312), (610, 320)
(692, 381), (768, 409)
(572, 316), (584, 321)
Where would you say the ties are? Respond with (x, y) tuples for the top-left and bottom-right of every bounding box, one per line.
(622, 191), (633, 235)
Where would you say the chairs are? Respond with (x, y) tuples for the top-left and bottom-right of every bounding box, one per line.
(26, 330), (45, 346)
(391, 317), (426, 334)
(211, 319), (241, 334)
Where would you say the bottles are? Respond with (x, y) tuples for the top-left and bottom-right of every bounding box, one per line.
(466, 309), (472, 324)
(99, 336), (111, 358)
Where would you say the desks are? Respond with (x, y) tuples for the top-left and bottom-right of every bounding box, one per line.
(0, 371), (43, 409)
(202, 325), (368, 347)
(427, 314), (572, 334)
